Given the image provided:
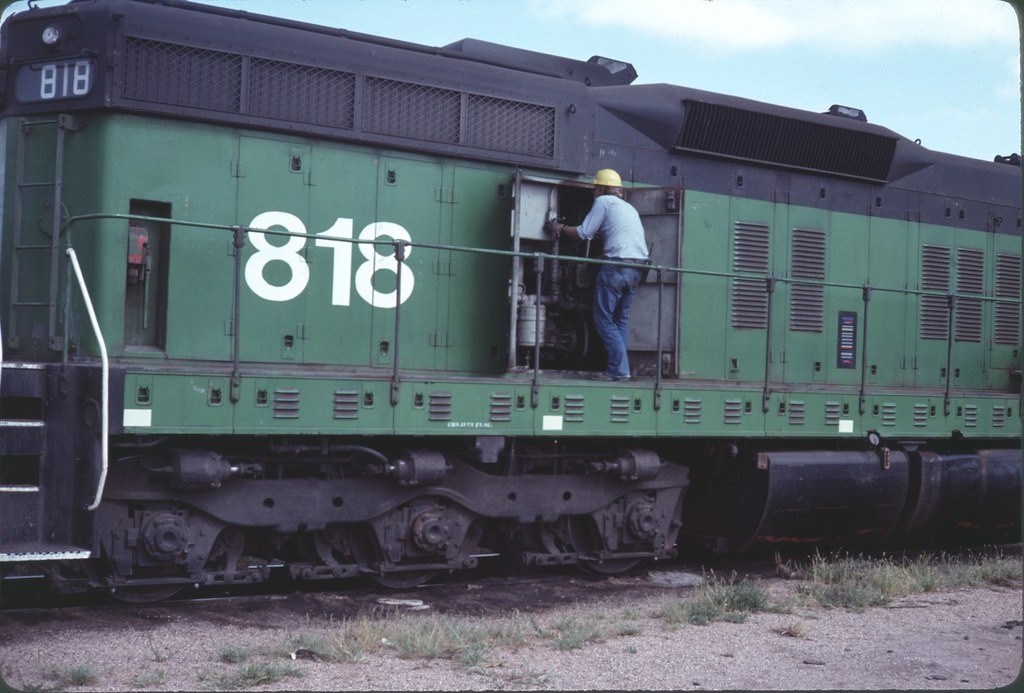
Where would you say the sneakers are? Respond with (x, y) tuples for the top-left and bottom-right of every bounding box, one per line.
(591, 372), (630, 382)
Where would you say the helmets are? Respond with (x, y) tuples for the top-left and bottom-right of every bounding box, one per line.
(592, 169), (623, 187)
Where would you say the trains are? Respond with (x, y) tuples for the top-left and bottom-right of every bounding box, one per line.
(0, 0), (1024, 601)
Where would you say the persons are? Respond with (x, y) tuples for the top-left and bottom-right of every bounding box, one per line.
(543, 169), (650, 382)
